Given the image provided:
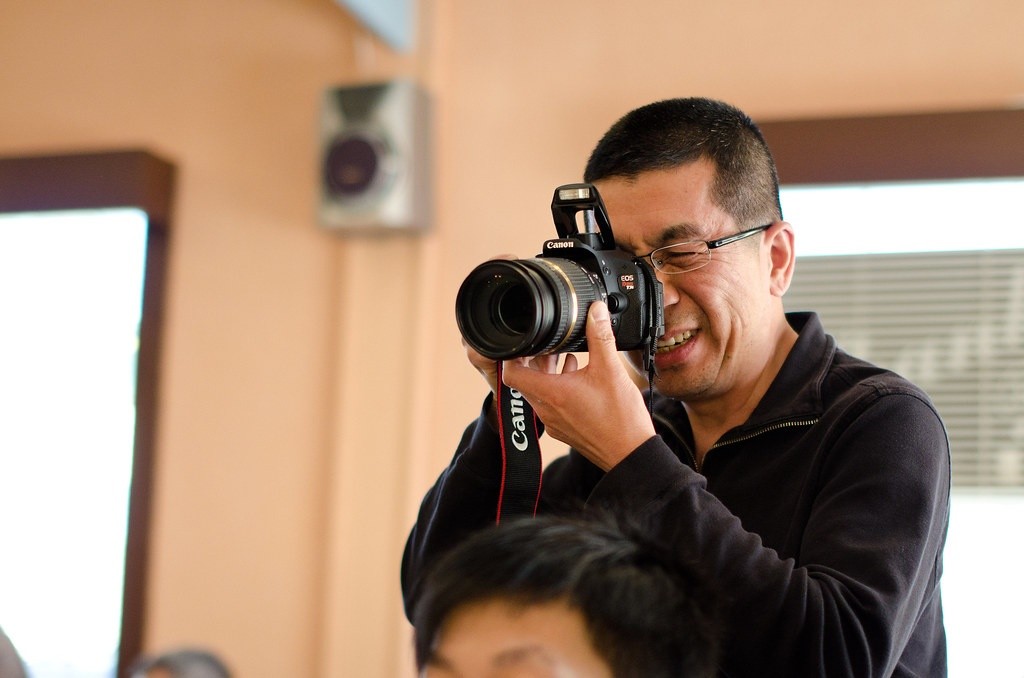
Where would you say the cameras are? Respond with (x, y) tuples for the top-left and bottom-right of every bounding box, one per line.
(455, 183), (664, 360)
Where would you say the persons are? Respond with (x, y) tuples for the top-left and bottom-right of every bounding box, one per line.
(415, 516), (692, 678)
(400, 96), (951, 678)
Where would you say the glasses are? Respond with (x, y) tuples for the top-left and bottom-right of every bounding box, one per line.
(632, 223), (774, 275)
